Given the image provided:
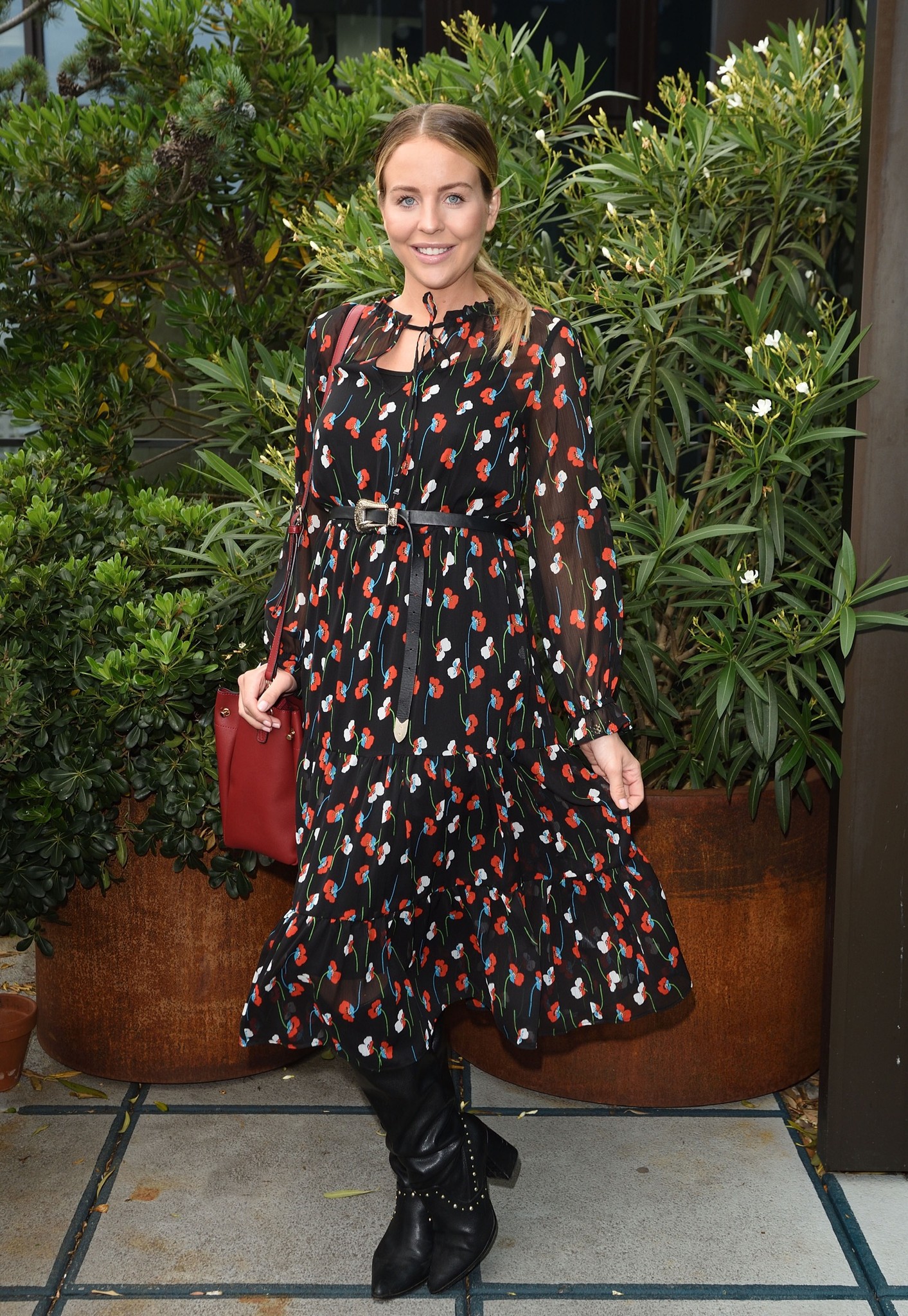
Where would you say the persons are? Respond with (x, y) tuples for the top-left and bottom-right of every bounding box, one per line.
(239, 103), (695, 1300)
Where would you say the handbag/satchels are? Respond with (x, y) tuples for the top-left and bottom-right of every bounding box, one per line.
(214, 687), (304, 866)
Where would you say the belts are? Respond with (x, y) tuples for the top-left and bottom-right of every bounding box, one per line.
(325, 499), (518, 741)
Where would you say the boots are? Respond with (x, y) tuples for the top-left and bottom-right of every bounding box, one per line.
(372, 1130), (458, 1299)
(310, 974), (519, 1294)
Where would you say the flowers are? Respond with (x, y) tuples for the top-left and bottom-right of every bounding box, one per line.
(535, 16), (907, 835)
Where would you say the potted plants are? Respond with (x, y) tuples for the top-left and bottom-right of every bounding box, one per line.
(1, 2), (357, 1084)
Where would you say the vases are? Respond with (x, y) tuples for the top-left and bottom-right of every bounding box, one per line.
(448, 770), (836, 1112)
(0, 993), (37, 1093)
(0, 1042), (0, 1043)
